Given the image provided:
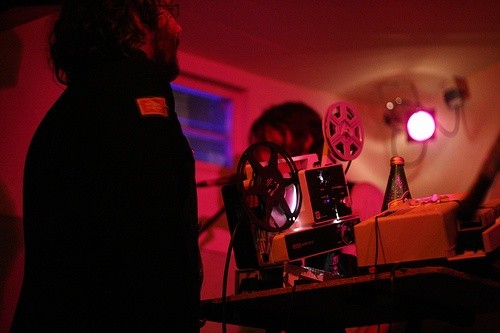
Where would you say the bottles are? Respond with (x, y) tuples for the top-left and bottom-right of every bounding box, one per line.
(382, 155), (411, 211)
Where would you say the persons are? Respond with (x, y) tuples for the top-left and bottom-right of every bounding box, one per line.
(249, 101), (385, 333)
(10, 0), (203, 333)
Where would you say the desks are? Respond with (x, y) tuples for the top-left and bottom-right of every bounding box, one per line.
(200, 266), (500, 333)
